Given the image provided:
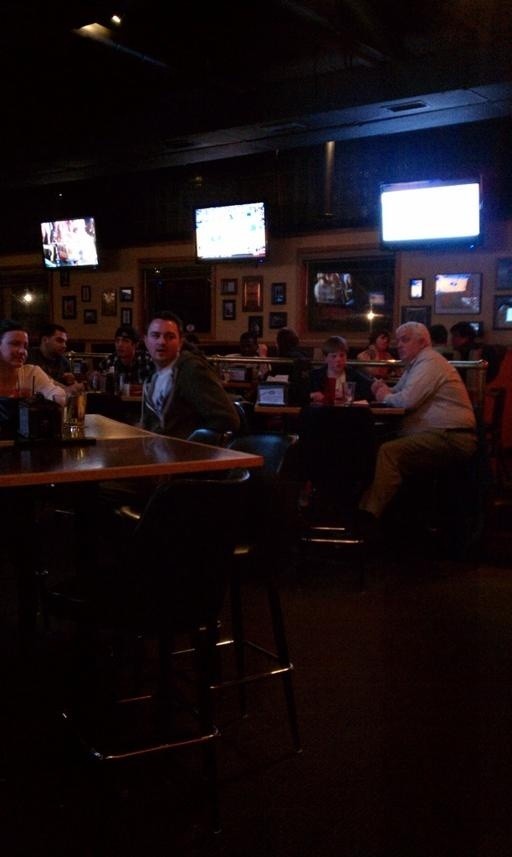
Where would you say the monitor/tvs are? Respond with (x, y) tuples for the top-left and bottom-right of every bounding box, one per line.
(39, 213), (103, 272)
(378, 173), (488, 251)
(192, 200), (269, 265)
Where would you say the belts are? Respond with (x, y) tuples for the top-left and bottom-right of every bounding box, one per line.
(434, 426), (474, 435)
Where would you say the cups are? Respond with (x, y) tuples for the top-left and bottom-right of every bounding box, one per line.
(63, 390), (86, 431)
(321, 375), (336, 405)
(342, 382), (355, 405)
(89, 369), (130, 395)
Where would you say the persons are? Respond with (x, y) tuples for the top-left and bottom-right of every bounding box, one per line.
(98, 326), (155, 384)
(0, 320), (73, 407)
(431, 321), (483, 380)
(276, 327), (306, 357)
(360, 321), (479, 531)
(134, 311), (240, 445)
(314, 272), (352, 304)
(238, 333), (263, 357)
(292, 336), (370, 515)
(358, 331), (401, 380)
(29, 324), (77, 386)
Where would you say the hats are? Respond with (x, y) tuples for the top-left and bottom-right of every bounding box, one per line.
(114, 326), (137, 342)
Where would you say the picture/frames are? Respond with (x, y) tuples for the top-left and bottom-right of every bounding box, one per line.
(401, 257), (512, 337)
(220, 275), (287, 337)
(60, 268), (134, 325)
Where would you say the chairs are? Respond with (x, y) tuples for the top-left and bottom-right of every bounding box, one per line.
(42, 479), (271, 857)
